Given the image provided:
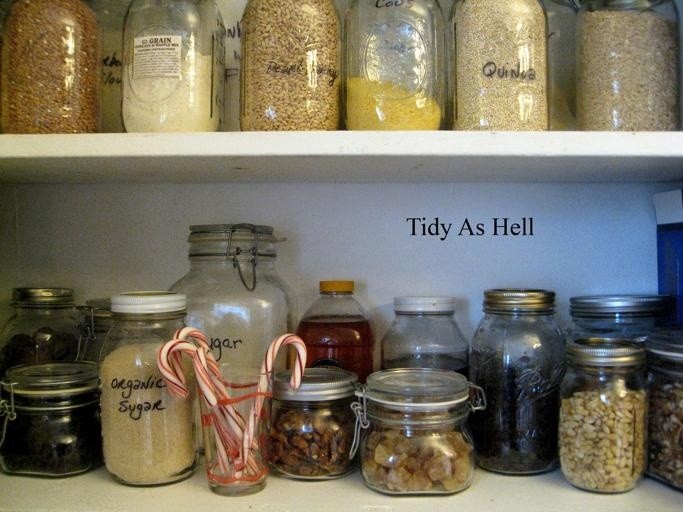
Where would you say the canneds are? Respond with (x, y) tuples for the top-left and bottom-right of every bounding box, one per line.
(0, 287), (199, 495)
(556, 291), (683, 495)
(375, 295), (469, 375)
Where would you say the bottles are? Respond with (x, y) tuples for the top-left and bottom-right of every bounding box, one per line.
(296, 280), (376, 385)
(2, 285), (96, 370)
(380, 294), (471, 383)
(559, 337), (650, 496)
(567, 294), (670, 345)
(644, 330), (682, 494)
(240, 0), (342, 132)
(100, 289), (205, 489)
(120, 0), (227, 132)
(346, 0), (449, 130)
(166, 221), (296, 456)
(2, 363), (103, 479)
(574, 0), (682, 131)
(0, 0), (104, 135)
(82, 294), (110, 362)
(450, 0), (551, 131)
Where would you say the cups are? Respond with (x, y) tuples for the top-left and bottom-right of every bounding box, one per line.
(196, 364), (271, 498)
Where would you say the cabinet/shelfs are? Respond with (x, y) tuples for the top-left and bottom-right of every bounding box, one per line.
(0, 129), (683, 512)
